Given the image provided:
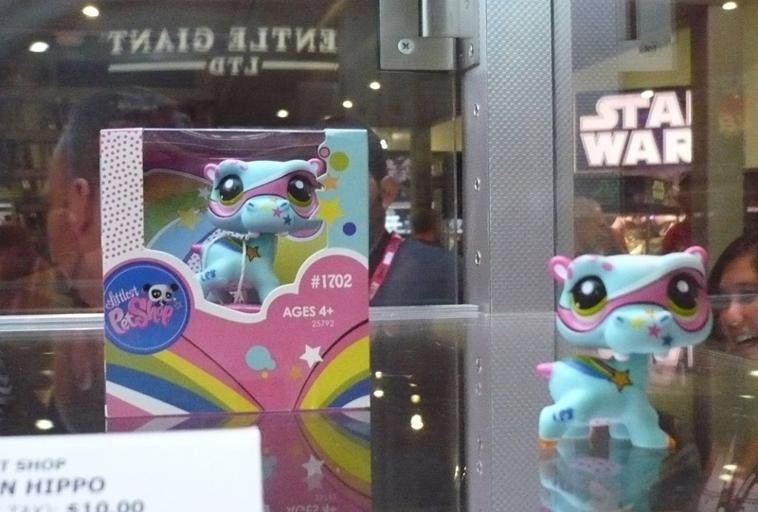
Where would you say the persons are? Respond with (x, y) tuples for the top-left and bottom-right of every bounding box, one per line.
(570, 189), (628, 261)
(654, 228), (757, 512)
(656, 170), (693, 254)
(0, 224), (34, 436)
(311, 113), (464, 509)
(35, 70), (212, 433)
(404, 204), (446, 249)
